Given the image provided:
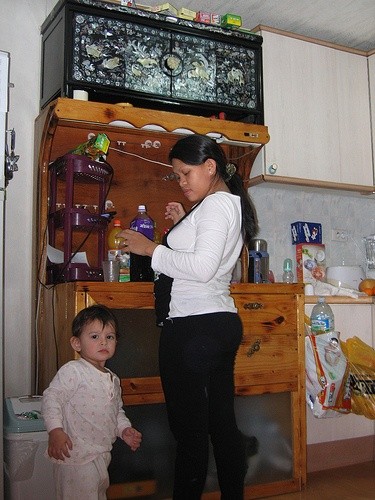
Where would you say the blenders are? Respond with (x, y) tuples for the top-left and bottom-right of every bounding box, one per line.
(363, 234), (375, 280)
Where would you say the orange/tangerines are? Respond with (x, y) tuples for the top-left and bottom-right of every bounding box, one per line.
(364, 287), (375, 296)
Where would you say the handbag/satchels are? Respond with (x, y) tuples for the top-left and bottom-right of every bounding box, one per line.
(303, 324), (375, 418)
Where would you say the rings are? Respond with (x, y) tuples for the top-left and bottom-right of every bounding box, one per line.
(124, 240), (126, 246)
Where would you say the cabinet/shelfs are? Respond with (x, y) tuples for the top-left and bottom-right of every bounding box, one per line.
(31, 0), (375, 500)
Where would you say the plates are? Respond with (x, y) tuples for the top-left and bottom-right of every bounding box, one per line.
(171, 128), (196, 134)
(107, 120), (136, 129)
(204, 132), (228, 140)
(140, 124), (167, 132)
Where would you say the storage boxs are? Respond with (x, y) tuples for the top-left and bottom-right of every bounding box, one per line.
(211, 12), (221, 27)
(177, 8), (196, 21)
(153, 3), (177, 16)
(3, 394), (56, 500)
(71, 133), (111, 160)
(291, 221), (322, 245)
(194, 10), (211, 24)
(222, 14), (242, 29)
(296, 243), (327, 283)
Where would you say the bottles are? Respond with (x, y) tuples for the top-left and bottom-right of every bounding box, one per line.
(248, 238), (269, 283)
(282, 257), (294, 283)
(129, 205), (154, 282)
(108, 219), (128, 273)
(310, 298), (335, 336)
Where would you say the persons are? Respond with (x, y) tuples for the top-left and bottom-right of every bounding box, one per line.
(40, 304), (142, 500)
(113, 133), (261, 500)
(323, 338), (342, 367)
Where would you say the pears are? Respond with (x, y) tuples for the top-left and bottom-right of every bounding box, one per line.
(359, 278), (375, 291)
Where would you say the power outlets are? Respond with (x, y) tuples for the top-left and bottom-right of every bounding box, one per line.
(332, 230), (347, 241)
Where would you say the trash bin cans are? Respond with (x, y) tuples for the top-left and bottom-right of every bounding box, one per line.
(3, 395), (57, 500)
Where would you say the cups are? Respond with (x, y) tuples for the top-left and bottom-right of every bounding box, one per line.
(304, 283), (315, 296)
(102, 260), (121, 283)
(72, 91), (88, 101)
